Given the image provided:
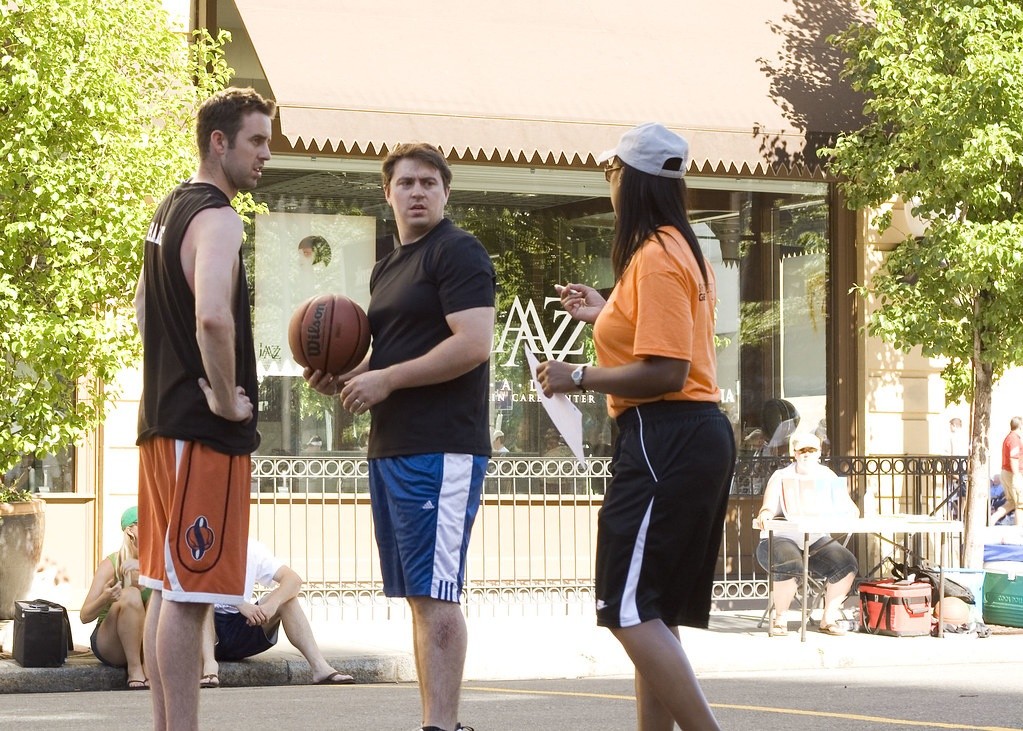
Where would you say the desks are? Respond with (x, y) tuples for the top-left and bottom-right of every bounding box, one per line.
(752, 512), (964, 641)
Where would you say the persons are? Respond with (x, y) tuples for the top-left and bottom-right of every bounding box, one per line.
(133, 87), (277, 731)
(293, 144), (498, 731)
(79, 507), (153, 689)
(198, 539), (358, 687)
(755, 434), (860, 637)
(537, 122), (738, 731)
(987, 416), (1022, 526)
(945, 418), (968, 521)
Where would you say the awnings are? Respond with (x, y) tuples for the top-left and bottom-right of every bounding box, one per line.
(233, 2), (840, 178)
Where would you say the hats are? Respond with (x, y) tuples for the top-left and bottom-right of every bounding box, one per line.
(121, 506), (138, 530)
(793, 434), (820, 450)
(598, 122), (689, 178)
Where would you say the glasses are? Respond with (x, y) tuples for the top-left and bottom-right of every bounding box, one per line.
(605, 166), (621, 182)
(799, 448), (817, 454)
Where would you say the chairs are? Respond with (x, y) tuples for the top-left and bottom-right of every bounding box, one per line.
(758, 533), (853, 633)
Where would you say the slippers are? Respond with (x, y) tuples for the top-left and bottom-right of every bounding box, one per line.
(773, 625), (788, 635)
(314, 672), (355, 684)
(128, 679), (150, 689)
(200, 675), (218, 687)
(819, 625), (846, 635)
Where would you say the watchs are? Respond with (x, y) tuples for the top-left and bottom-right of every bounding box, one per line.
(571, 365), (587, 393)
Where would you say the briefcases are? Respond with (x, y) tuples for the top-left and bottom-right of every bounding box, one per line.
(858, 581), (932, 636)
(12, 599), (74, 667)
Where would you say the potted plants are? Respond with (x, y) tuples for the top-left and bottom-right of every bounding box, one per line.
(0, 0), (271, 622)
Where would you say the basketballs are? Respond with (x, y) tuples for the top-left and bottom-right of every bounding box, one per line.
(288, 291), (373, 374)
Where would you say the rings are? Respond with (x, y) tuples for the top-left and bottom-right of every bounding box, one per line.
(757, 521), (758, 523)
(355, 398), (361, 404)
